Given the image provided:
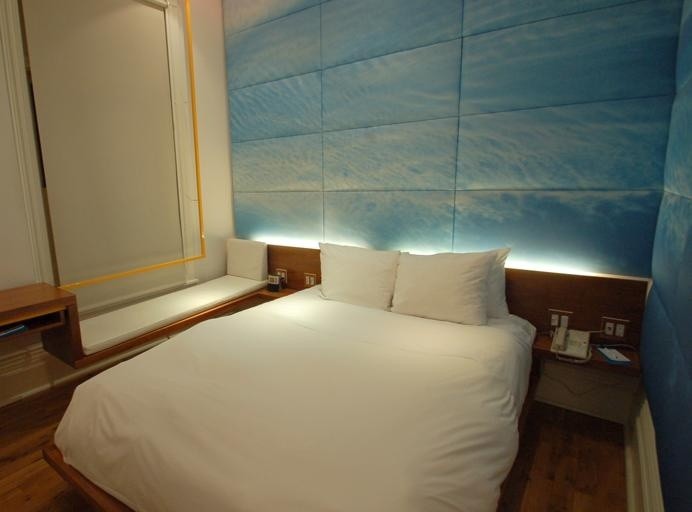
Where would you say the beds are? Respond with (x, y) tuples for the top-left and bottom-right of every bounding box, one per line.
(43, 240), (542, 511)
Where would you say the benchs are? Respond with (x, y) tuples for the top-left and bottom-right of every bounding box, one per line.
(69, 237), (297, 369)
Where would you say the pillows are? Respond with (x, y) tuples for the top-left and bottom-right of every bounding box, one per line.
(314, 243), (398, 312)
(389, 245), (496, 326)
(487, 246), (513, 321)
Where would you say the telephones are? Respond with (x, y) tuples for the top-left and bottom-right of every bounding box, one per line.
(550, 327), (591, 360)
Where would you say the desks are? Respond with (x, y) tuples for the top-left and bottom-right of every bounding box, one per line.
(528, 333), (643, 376)
(257, 285), (297, 303)
